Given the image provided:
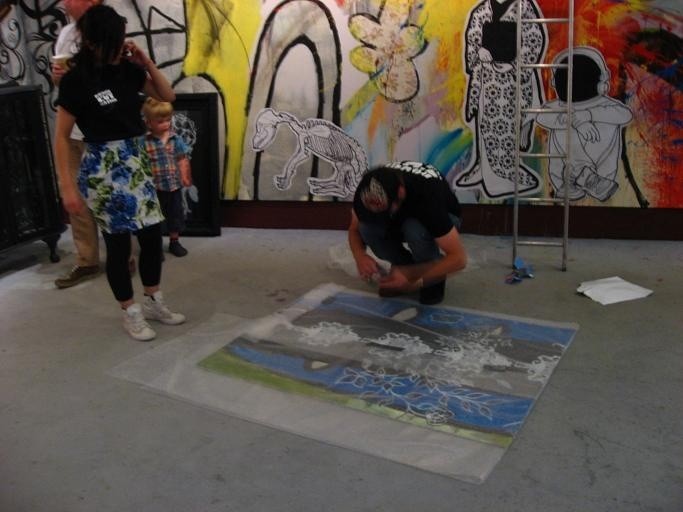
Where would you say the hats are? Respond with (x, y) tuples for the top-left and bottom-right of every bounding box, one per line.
(350, 167), (398, 223)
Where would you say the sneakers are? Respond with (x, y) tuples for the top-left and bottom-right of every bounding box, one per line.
(54, 262), (101, 289)
(376, 282), (408, 301)
(167, 237), (187, 258)
(416, 275), (446, 306)
(140, 291), (185, 327)
(555, 165), (620, 203)
(116, 302), (160, 344)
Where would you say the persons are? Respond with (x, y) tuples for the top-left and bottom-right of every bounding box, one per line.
(52, 0), (109, 291)
(345, 161), (465, 307)
(55, 4), (186, 342)
(138, 97), (194, 259)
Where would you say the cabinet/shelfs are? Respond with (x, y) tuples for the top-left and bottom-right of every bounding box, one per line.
(0, 85), (65, 263)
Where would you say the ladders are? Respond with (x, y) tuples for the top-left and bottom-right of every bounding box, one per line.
(511, 0), (574, 271)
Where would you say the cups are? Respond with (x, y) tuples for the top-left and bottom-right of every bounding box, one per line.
(52, 55), (66, 72)
